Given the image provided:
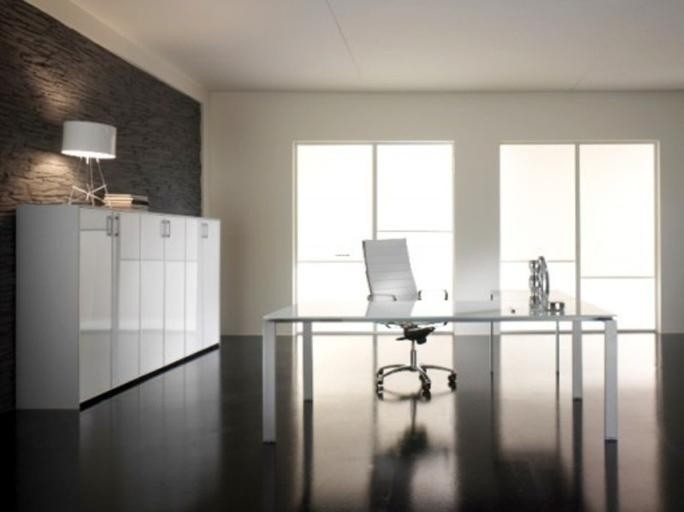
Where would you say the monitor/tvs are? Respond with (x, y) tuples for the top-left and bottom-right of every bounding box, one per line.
(101, 193), (151, 211)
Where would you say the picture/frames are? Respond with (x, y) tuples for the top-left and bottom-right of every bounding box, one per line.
(260, 299), (618, 446)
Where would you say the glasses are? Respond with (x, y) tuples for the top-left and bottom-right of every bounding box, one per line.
(61, 119), (117, 208)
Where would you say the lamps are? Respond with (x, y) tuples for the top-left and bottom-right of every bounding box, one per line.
(361, 238), (456, 394)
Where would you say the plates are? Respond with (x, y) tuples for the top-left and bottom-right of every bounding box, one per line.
(14, 201), (221, 411)
